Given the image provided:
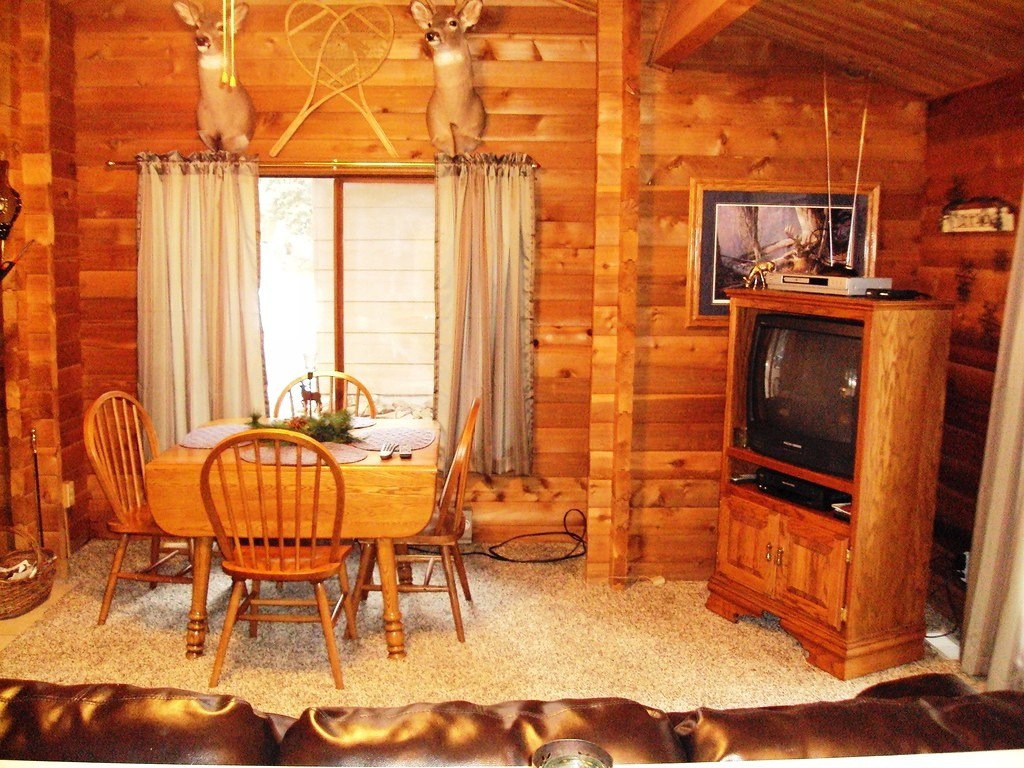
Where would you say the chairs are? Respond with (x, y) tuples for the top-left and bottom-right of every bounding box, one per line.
(339, 393), (483, 644)
(268, 369), (381, 423)
(82, 390), (203, 627)
(198, 428), (359, 692)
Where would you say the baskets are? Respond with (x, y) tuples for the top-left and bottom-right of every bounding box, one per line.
(0, 524), (57, 622)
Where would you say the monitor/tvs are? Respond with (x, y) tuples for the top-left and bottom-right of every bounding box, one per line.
(747, 314), (865, 480)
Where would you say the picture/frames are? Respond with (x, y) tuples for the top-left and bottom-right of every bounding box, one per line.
(681, 175), (885, 336)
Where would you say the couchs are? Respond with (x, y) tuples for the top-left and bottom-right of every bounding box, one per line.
(0, 674), (1024, 766)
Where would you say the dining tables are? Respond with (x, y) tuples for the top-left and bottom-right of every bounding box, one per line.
(142, 418), (441, 665)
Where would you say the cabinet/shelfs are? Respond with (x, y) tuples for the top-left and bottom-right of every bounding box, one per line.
(700, 282), (953, 681)
